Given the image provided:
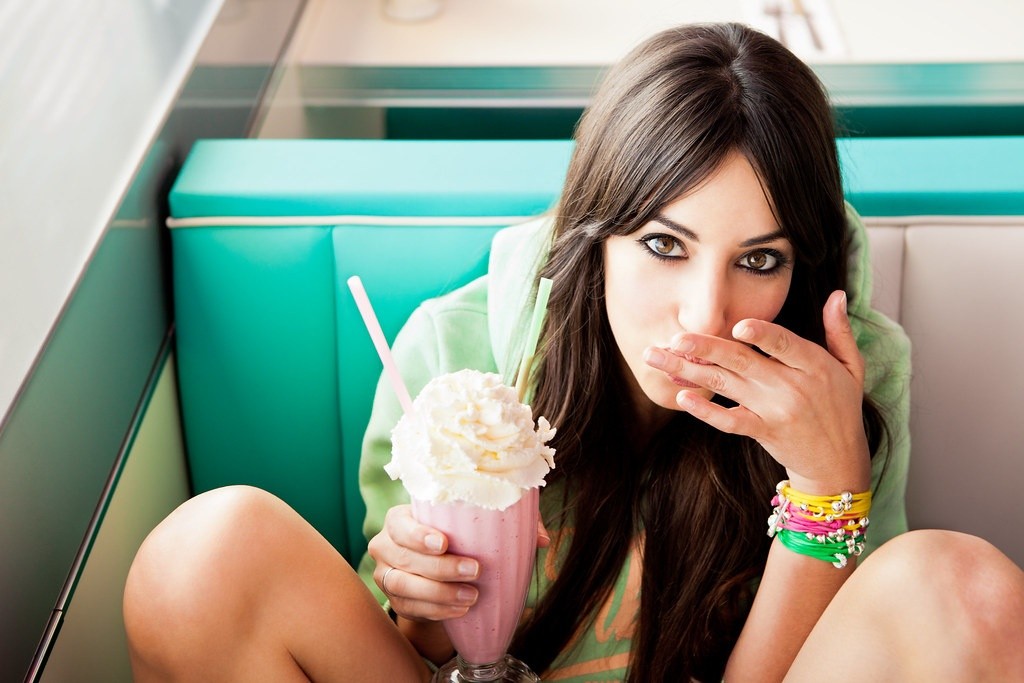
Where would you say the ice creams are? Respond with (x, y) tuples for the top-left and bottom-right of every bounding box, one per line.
(384, 370), (557, 665)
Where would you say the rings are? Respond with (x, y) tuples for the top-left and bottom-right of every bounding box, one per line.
(381, 568), (399, 598)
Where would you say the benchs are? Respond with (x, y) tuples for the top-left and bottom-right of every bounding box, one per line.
(161, 128), (1024, 569)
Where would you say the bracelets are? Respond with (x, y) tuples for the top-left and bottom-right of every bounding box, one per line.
(766, 479), (872, 569)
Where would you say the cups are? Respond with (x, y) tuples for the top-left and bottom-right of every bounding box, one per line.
(410, 486), (541, 683)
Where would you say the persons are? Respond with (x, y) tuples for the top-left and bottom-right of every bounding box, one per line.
(119, 22), (1024, 683)
